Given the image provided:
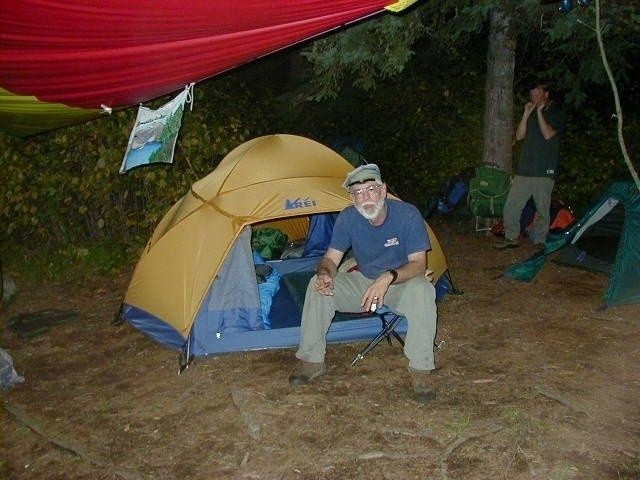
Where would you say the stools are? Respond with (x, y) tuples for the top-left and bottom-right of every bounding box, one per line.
(350, 304), (405, 366)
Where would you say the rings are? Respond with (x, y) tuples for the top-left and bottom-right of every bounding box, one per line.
(373, 296), (378, 300)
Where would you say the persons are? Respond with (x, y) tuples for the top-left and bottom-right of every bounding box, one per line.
(287, 163), (438, 401)
(492, 79), (567, 258)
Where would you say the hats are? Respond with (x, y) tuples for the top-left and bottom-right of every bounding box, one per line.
(341, 163), (383, 190)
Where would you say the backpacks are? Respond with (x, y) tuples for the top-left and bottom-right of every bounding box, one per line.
(433, 172), (471, 215)
(467, 163), (513, 220)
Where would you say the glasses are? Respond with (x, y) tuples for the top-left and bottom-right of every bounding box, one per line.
(347, 185), (382, 199)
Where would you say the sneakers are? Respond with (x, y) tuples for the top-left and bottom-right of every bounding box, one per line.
(284, 359), (329, 386)
(493, 239), (520, 251)
(407, 364), (437, 395)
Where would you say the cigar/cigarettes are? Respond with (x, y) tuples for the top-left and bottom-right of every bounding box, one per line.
(315, 281), (331, 291)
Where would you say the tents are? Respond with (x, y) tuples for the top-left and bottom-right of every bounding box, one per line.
(495, 177), (639, 312)
(112, 133), (466, 379)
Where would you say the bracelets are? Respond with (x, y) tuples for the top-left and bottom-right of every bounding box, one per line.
(385, 268), (398, 285)
